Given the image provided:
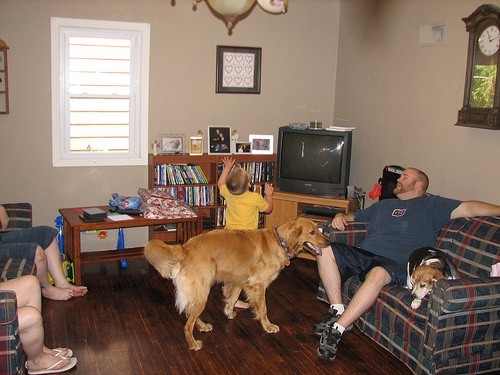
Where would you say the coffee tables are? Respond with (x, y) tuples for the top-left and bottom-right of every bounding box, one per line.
(58, 201), (200, 288)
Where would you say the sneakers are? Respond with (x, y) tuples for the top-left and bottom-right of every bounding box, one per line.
(316, 321), (342, 361)
(312, 306), (354, 335)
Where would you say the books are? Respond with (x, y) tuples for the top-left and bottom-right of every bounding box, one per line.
(153, 163), (209, 185)
(218, 208), (226, 227)
(218, 162), (273, 184)
(259, 211), (264, 225)
(217, 185), (267, 206)
(154, 186), (214, 207)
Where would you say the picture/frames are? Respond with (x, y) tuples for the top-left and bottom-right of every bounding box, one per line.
(189, 136), (204, 156)
(157, 134), (187, 156)
(215, 45), (262, 95)
(208, 126), (232, 155)
(234, 142), (252, 154)
(248, 134), (273, 155)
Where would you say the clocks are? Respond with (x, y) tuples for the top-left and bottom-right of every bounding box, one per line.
(454, 4), (500, 130)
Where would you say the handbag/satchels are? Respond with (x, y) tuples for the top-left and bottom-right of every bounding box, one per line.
(138, 188), (198, 219)
(108, 193), (144, 213)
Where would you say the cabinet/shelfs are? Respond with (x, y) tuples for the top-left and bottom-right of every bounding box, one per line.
(264, 190), (362, 261)
(148, 153), (278, 245)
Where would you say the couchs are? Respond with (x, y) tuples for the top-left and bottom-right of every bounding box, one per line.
(317, 192), (500, 375)
(0, 202), (38, 375)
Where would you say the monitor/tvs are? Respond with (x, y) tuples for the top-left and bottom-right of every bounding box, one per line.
(276, 126), (352, 199)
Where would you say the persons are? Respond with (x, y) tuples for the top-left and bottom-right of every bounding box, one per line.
(253, 140), (269, 150)
(311, 168), (500, 360)
(0, 206), (88, 301)
(237, 145), (249, 152)
(0, 275), (78, 375)
(163, 140), (180, 152)
(218, 156), (274, 308)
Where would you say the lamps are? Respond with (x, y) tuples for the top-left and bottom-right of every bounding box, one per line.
(170, 0), (289, 37)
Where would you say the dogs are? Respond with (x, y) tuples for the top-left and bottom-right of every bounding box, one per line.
(144, 215), (332, 352)
(407, 246), (459, 310)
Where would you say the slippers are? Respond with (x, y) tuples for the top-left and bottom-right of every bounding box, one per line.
(28, 357), (77, 374)
(25, 348), (73, 369)
